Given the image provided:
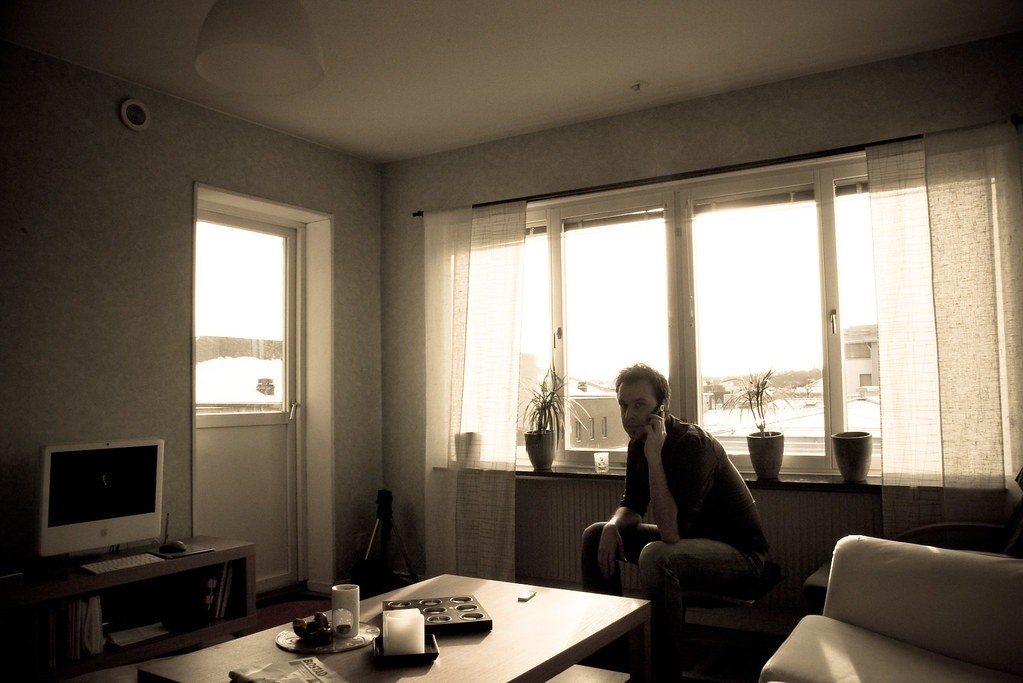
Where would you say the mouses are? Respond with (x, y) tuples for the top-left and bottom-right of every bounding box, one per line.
(159, 540), (187, 554)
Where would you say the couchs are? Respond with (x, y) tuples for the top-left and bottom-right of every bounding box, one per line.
(758, 527), (1023, 683)
(802, 521), (1005, 616)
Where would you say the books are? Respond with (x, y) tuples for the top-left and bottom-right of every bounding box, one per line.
(43, 593), (105, 667)
(197, 561), (233, 619)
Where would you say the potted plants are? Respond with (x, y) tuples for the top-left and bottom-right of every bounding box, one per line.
(512, 367), (591, 470)
(719, 369), (796, 476)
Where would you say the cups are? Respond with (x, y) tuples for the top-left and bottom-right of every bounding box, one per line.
(331, 583), (359, 637)
(832, 432), (872, 481)
(594, 452), (610, 472)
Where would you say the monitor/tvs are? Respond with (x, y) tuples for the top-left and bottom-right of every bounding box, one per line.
(38, 437), (165, 566)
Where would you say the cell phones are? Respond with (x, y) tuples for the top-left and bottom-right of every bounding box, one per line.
(650, 406), (666, 428)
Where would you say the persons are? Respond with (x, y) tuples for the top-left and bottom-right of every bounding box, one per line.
(580, 362), (771, 683)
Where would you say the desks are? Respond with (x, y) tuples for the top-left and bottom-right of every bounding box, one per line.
(0, 536), (257, 683)
(133, 574), (653, 683)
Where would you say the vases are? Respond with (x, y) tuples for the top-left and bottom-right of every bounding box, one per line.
(831, 432), (873, 480)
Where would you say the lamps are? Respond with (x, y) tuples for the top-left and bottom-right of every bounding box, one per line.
(194, 0), (324, 98)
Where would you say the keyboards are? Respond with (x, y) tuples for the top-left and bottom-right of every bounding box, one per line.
(83, 552), (165, 574)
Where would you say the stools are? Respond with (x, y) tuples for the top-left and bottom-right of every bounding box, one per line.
(682, 582), (771, 629)
